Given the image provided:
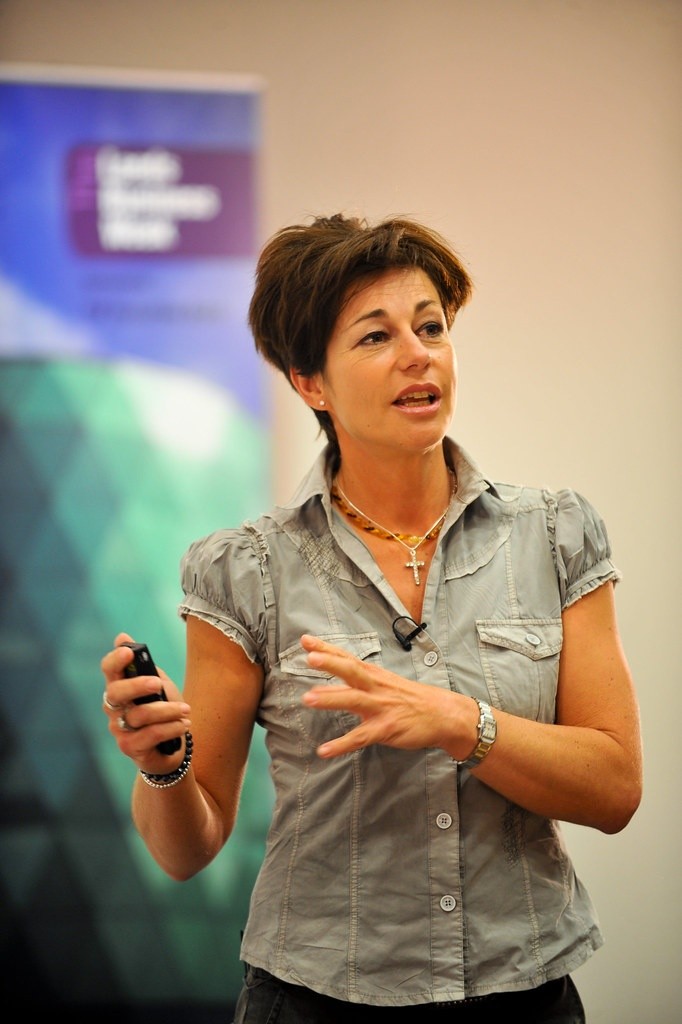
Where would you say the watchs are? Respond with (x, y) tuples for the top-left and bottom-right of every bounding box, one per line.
(449, 696), (497, 768)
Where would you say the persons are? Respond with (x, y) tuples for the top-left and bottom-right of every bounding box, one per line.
(100, 214), (642, 1024)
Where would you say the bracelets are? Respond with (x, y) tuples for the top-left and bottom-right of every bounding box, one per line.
(140, 733), (193, 789)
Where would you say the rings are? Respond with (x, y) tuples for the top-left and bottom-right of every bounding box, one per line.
(103, 692), (121, 711)
(117, 713), (133, 732)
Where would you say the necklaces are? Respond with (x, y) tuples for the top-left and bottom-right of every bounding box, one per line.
(331, 468), (458, 586)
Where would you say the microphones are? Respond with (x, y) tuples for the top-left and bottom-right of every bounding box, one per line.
(393, 628), (413, 652)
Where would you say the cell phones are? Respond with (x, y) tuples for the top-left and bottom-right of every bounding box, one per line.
(119, 641), (182, 755)
